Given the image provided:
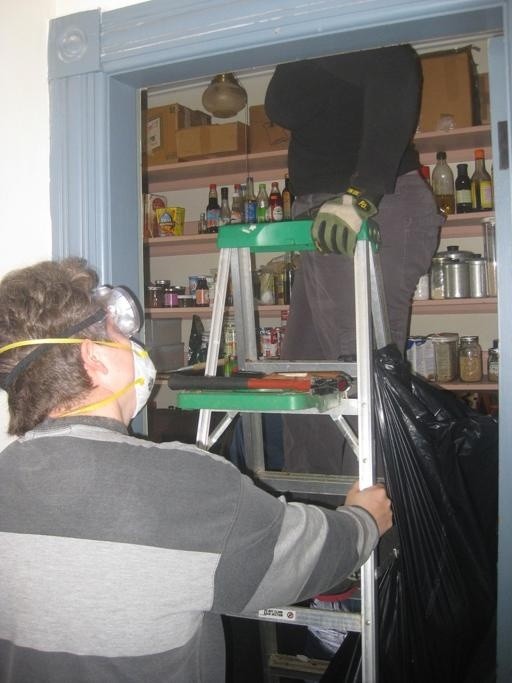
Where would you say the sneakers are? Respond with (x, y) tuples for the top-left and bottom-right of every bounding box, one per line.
(305, 599), (356, 657)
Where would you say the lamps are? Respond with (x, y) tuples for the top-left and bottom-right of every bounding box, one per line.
(197, 68), (253, 122)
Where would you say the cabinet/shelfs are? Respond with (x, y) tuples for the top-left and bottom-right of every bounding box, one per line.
(141, 121), (501, 398)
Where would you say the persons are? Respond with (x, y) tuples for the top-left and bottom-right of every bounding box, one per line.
(0, 260), (393, 681)
(256, 45), (446, 647)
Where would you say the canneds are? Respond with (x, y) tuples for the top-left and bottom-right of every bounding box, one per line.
(146, 276), (206, 308)
(406, 337), (436, 382)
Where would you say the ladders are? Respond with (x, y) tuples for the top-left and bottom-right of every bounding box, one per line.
(175, 217), (391, 683)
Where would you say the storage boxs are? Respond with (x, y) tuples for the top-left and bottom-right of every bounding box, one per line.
(409, 38), (482, 136)
(139, 96), (219, 165)
(244, 99), (295, 155)
(173, 114), (251, 162)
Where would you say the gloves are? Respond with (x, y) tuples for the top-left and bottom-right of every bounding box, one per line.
(313, 190), (382, 258)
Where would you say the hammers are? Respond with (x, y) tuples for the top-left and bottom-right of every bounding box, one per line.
(167, 374), (349, 397)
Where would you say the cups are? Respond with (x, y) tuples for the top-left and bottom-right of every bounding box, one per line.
(412, 271), (429, 300)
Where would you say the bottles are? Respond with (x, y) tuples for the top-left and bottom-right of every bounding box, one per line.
(283, 250), (295, 304)
(454, 162), (472, 212)
(470, 148), (492, 212)
(195, 276), (210, 305)
(427, 331), (499, 383)
(197, 172), (295, 233)
(430, 242), (488, 299)
(432, 151), (455, 214)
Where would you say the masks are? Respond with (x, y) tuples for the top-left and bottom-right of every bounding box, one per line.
(130, 339), (159, 413)
